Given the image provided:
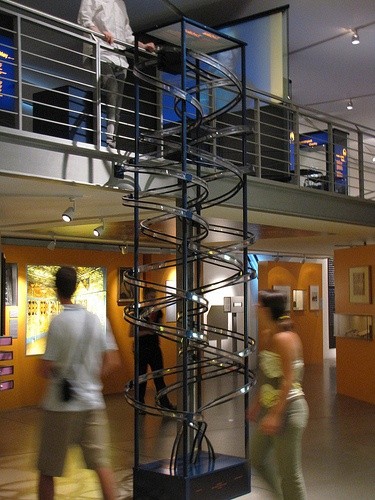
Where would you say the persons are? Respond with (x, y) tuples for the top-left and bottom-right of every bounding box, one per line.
(77, 0), (157, 146)
(128, 287), (176, 417)
(245, 289), (310, 500)
(33, 266), (124, 500)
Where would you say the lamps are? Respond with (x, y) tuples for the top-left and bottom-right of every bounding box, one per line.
(122, 241), (128, 254)
(352, 29), (359, 44)
(93, 217), (105, 236)
(47, 232), (56, 250)
(62, 198), (75, 222)
(346, 98), (352, 110)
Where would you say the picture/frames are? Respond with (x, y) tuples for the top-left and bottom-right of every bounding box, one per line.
(348, 265), (372, 304)
(117, 266), (134, 302)
